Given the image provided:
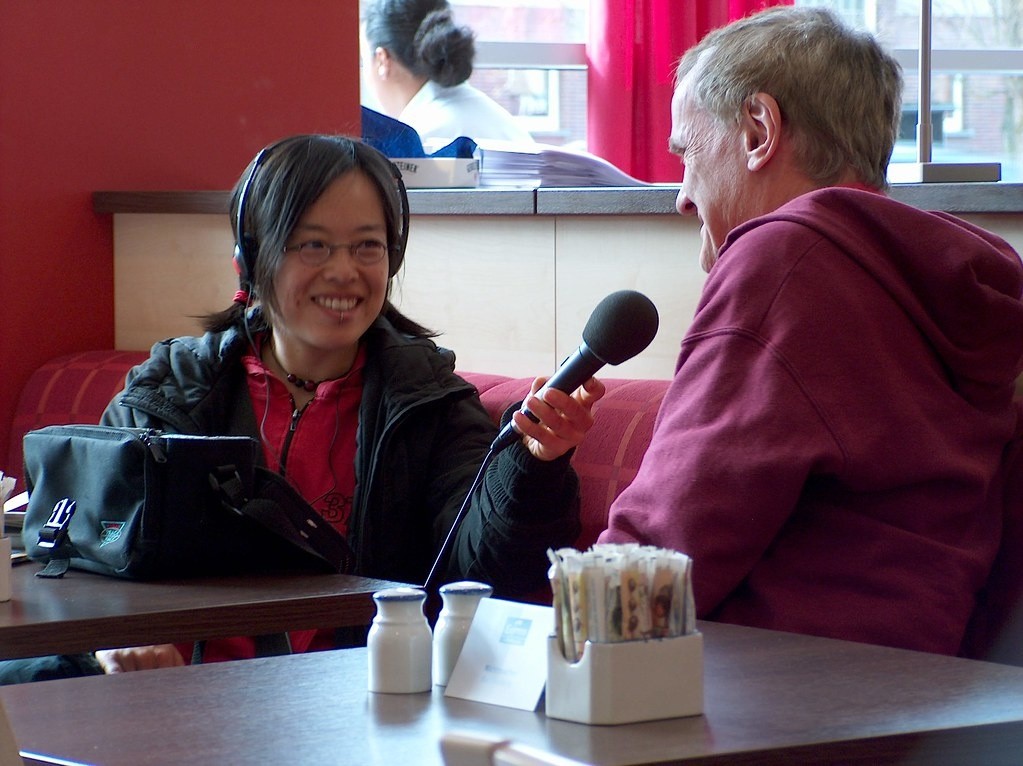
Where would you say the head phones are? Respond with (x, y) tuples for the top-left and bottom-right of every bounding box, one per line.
(234, 132), (409, 285)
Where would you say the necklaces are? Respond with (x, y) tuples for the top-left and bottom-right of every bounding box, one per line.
(269, 337), (331, 391)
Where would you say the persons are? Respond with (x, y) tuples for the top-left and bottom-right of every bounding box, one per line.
(593, 8), (1023, 658)
(0, 132), (605, 686)
(360, 0), (531, 142)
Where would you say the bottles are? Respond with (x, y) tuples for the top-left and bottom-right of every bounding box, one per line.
(0, 536), (12, 601)
(366, 588), (434, 693)
(435, 581), (493, 687)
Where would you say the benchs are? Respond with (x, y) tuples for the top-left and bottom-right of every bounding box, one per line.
(5, 347), (1023, 668)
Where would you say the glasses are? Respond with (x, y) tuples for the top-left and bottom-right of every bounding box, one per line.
(280, 239), (401, 266)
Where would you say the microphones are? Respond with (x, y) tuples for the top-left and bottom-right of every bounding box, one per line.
(491, 291), (659, 452)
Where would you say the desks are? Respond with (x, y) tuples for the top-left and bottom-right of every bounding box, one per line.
(0, 559), (428, 666)
(0, 620), (1023, 766)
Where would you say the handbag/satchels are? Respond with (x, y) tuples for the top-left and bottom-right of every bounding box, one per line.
(21, 413), (371, 586)
